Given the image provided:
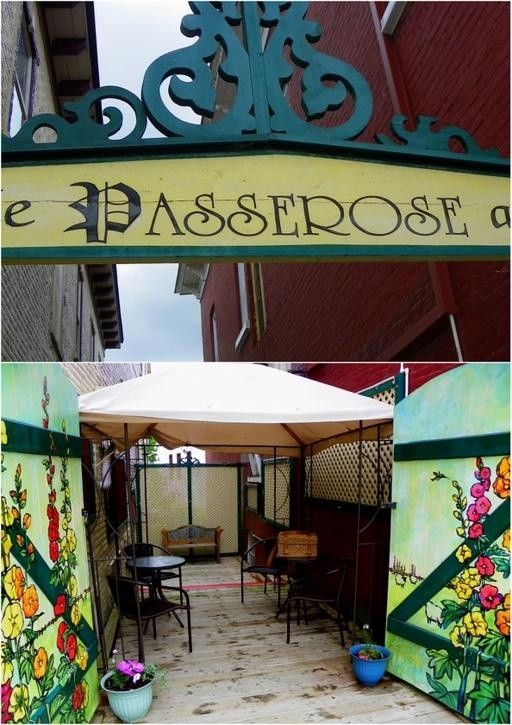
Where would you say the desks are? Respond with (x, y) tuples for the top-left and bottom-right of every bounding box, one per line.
(277, 555), (318, 625)
(126, 555), (185, 639)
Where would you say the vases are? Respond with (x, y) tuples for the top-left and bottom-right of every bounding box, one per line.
(101, 672), (154, 722)
(349, 644), (392, 687)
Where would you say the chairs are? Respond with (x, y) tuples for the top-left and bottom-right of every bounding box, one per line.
(286, 557), (354, 648)
(122, 543), (182, 604)
(240, 537), (284, 608)
(106, 574), (193, 653)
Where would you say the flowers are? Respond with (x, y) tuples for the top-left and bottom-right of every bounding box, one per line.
(423, 455), (511, 723)
(107, 647), (170, 693)
(359, 623), (383, 658)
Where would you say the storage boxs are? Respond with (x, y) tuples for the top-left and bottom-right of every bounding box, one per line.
(278, 530), (319, 558)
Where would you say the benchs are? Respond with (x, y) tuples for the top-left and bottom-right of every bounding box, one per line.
(161, 525), (224, 563)
